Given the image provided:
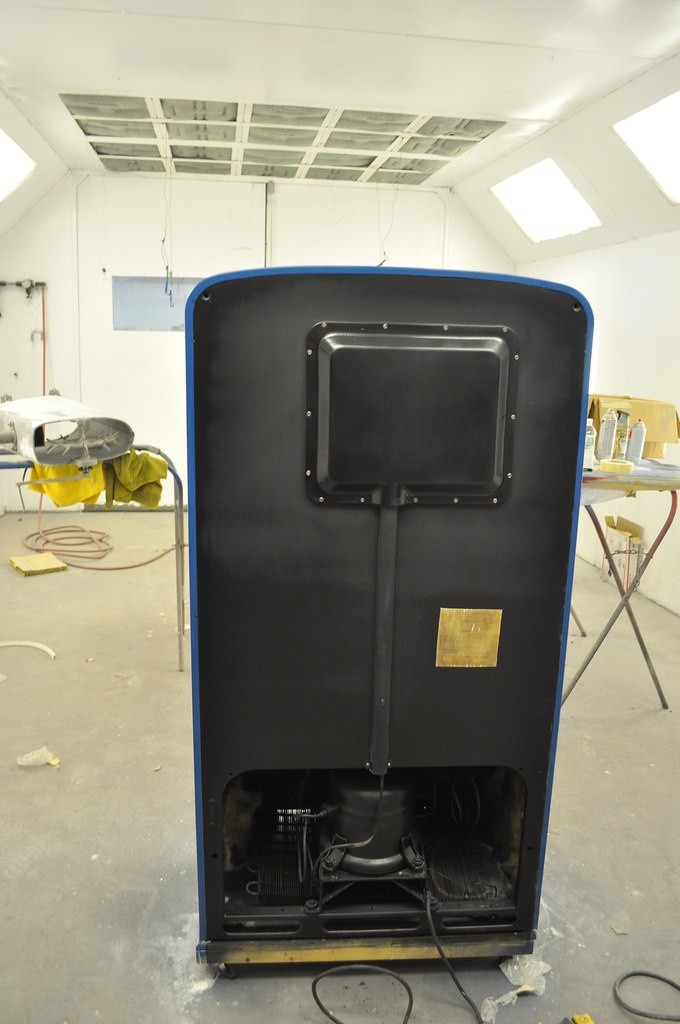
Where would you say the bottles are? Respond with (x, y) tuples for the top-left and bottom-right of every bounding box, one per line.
(582, 419), (597, 470)
(598, 408), (646, 465)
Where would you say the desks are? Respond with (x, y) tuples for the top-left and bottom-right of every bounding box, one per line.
(560, 455), (680, 711)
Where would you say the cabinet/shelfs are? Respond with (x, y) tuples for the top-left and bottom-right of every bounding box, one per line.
(0, 434), (187, 672)
